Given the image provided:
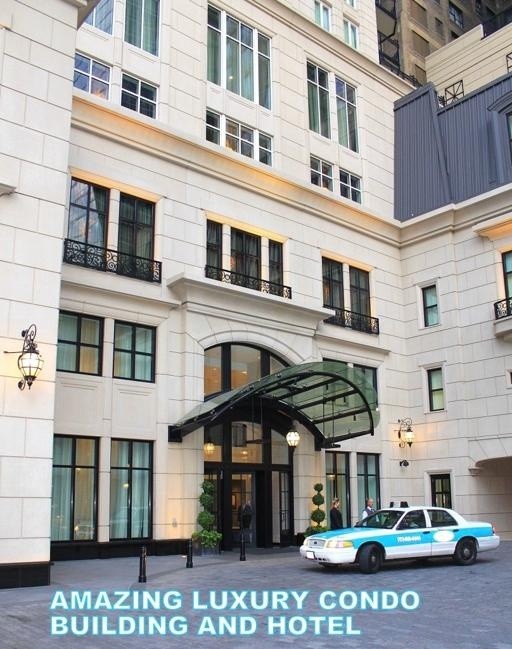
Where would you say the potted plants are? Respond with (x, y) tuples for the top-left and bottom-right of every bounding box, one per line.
(191, 479), (223, 558)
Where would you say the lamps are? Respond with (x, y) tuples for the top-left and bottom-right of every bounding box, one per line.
(285, 424), (300, 454)
(393, 415), (414, 448)
(4, 323), (45, 391)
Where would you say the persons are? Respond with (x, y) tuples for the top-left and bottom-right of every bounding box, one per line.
(329, 496), (344, 529)
(404, 513), (420, 528)
(361, 498), (380, 527)
(237, 496), (252, 543)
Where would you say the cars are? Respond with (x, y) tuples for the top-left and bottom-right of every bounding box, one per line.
(73, 506), (147, 540)
(300, 506), (500, 573)
